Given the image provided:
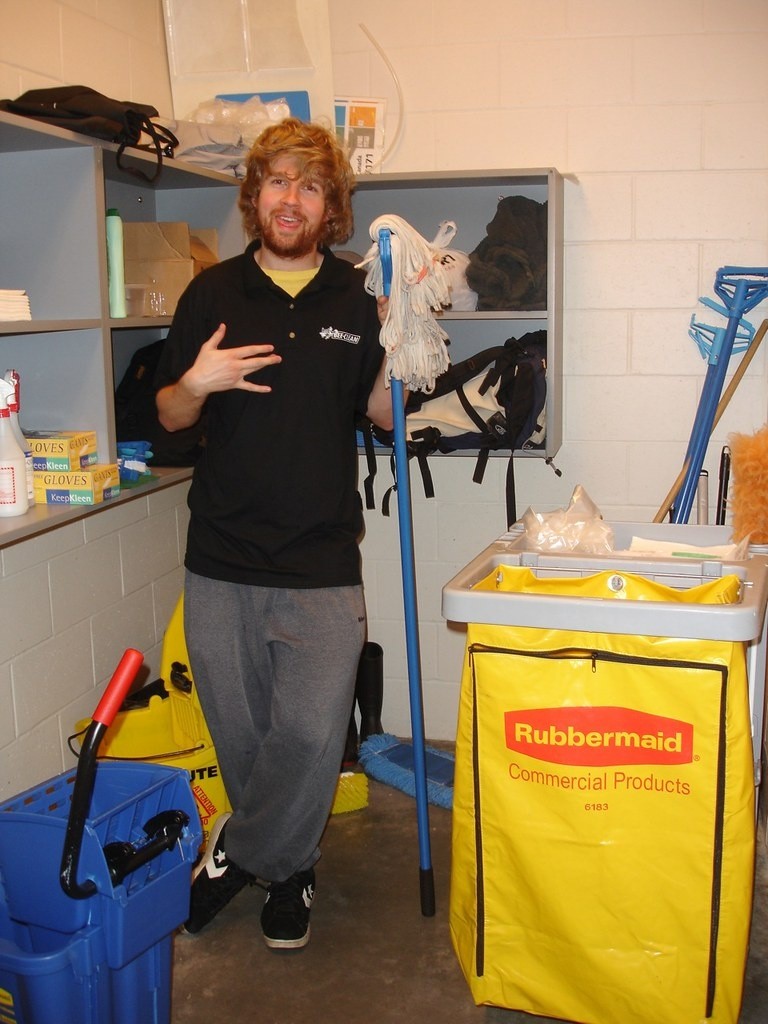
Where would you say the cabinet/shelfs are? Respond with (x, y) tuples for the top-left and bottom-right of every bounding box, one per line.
(326, 167), (564, 460)
(0, 109), (250, 550)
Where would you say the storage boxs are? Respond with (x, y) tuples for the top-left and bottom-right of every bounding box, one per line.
(0, 760), (205, 1023)
(23, 430), (98, 472)
(122, 222), (220, 317)
(33, 464), (121, 506)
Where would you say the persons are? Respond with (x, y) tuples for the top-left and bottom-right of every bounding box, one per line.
(153, 116), (411, 950)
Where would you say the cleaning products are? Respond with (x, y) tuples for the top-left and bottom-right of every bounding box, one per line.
(0, 366), (35, 518)
(106, 208), (128, 319)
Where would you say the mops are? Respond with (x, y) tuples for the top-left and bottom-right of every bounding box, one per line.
(350, 210), (466, 919)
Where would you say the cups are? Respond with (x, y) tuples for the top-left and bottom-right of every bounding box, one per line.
(124, 288), (146, 318)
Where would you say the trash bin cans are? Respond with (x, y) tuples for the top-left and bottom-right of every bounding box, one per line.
(439, 523), (768, 1024)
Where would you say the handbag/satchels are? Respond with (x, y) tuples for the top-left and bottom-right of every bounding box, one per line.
(0, 85), (180, 183)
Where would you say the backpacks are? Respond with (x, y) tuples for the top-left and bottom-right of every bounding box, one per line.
(355, 330), (549, 535)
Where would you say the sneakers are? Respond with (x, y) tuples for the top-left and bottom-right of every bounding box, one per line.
(261, 868), (317, 948)
(177, 811), (266, 936)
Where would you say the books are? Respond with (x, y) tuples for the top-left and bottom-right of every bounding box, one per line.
(0, 288), (32, 321)
